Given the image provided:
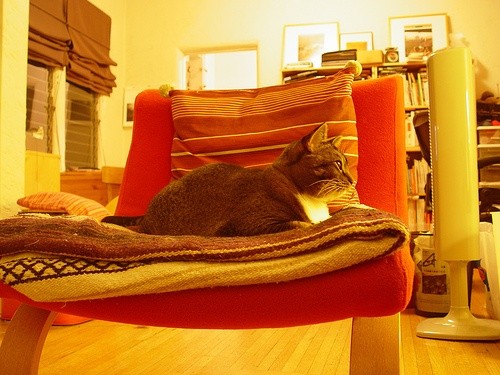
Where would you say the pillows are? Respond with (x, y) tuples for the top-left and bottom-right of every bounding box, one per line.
(158, 60), (361, 215)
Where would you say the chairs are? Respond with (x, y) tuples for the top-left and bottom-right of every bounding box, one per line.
(413, 111), (500, 223)
(0, 75), (417, 375)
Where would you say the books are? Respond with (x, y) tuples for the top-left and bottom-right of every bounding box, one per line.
(406, 152), (431, 196)
(407, 199), (432, 232)
(478, 129), (500, 146)
(478, 148), (500, 183)
(377, 66), (429, 108)
(283, 70), (371, 84)
(286, 49), (356, 68)
(405, 110), (420, 148)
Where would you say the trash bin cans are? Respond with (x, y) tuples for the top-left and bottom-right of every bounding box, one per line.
(410, 228), (473, 317)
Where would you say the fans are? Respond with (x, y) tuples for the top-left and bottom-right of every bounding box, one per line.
(415, 44), (500, 341)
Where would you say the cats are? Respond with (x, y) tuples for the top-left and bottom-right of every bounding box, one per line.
(100, 122), (355, 237)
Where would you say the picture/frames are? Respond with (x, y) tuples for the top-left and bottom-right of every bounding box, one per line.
(282, 13), (449, 65)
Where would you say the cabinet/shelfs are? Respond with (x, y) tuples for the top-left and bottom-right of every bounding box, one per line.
(286, 60), (500, 266)
